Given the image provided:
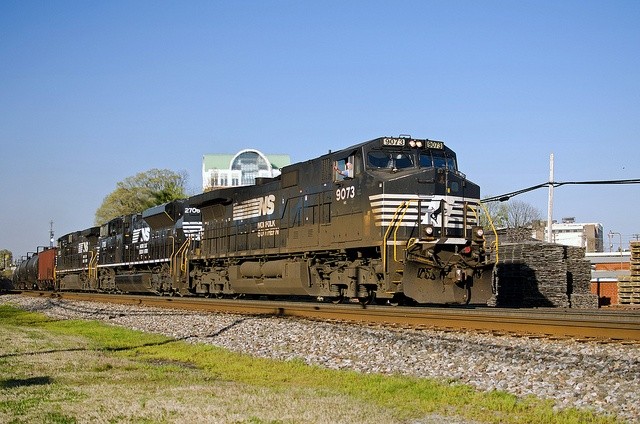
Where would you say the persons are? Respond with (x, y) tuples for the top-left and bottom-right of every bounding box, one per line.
(332, 162), (353, 178)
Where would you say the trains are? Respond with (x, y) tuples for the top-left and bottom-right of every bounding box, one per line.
(13, 135), (499, 306)
